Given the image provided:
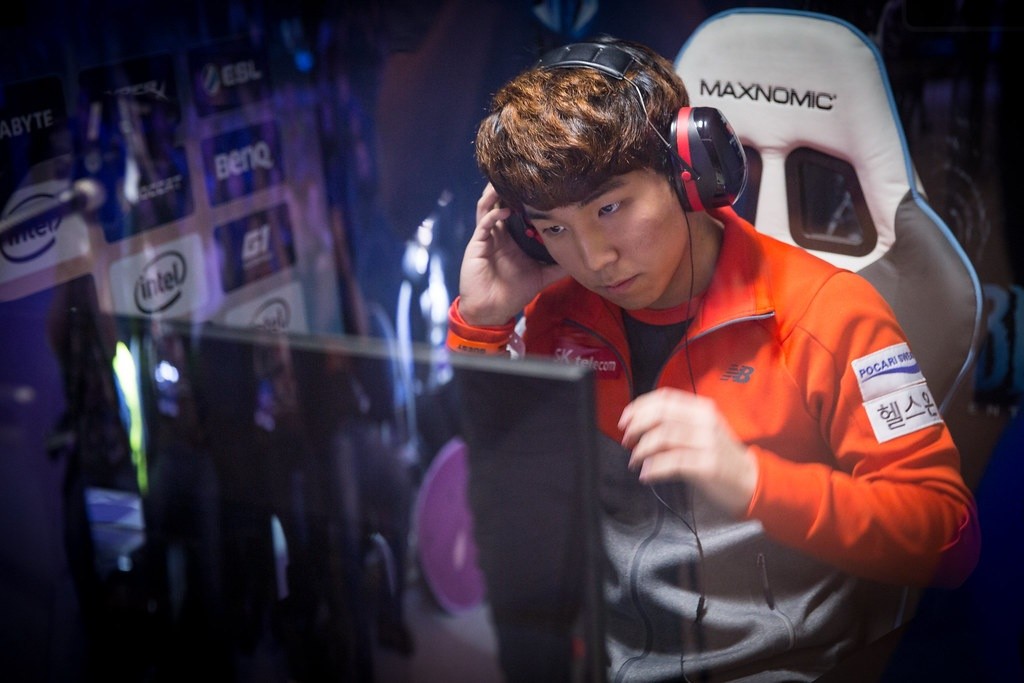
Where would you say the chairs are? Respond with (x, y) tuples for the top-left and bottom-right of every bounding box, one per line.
(672, 10), (986, 429)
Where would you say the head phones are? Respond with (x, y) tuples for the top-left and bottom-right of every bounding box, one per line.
(495, 42), (749, 267)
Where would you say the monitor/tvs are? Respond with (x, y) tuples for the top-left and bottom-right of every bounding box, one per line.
(53, 304), (613, 683)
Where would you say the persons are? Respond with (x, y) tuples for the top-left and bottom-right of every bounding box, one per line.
(443, 34), (981, 683)
